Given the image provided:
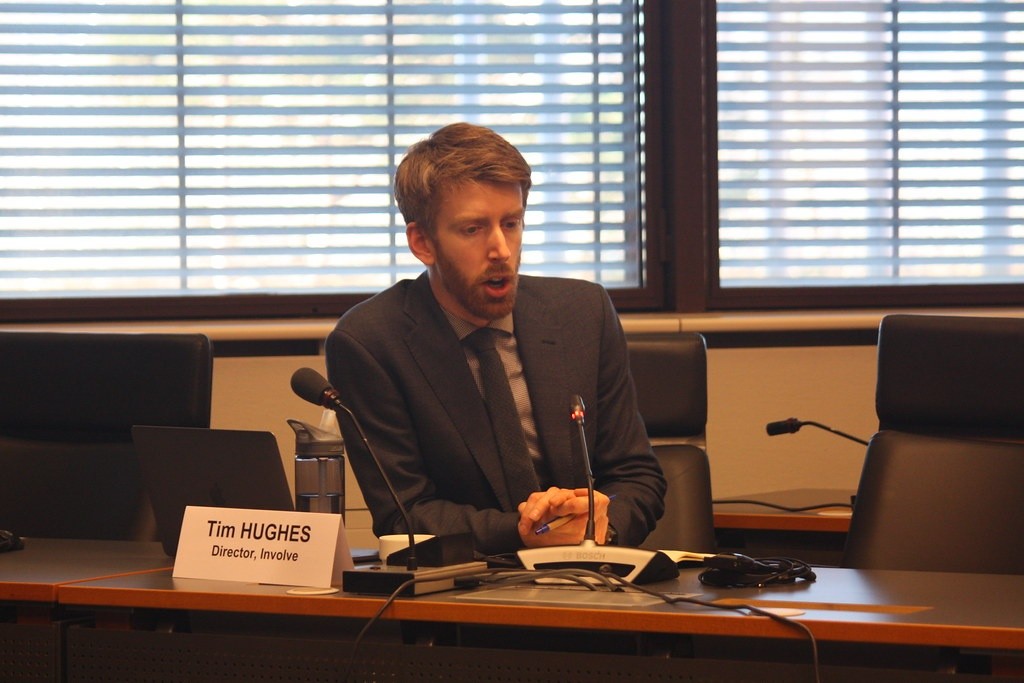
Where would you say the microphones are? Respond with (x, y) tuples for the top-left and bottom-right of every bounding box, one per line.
(767, 418), (869, 447)
(517, 392), (680, 586)
(291, 368), (488, 596)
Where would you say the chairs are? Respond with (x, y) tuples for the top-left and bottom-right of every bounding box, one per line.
(621, 330), (717, 558)
(842, 312), (1024, 579)
(1, 329), (219, 541)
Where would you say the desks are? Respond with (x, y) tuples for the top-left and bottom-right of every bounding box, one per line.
(0, 531), (1023, 683)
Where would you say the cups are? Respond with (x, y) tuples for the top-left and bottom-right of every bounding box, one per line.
(287, 418), (347, 530)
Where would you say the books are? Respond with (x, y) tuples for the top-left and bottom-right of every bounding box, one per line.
(655, 549), (723, 568)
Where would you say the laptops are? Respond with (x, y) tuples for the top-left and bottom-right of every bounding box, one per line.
(130, 426), (381, 564)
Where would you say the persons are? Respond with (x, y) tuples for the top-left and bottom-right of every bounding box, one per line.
(325, 120), (669, 567)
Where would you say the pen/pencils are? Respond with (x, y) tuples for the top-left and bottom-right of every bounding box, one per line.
(534, 494), (616, 536)
